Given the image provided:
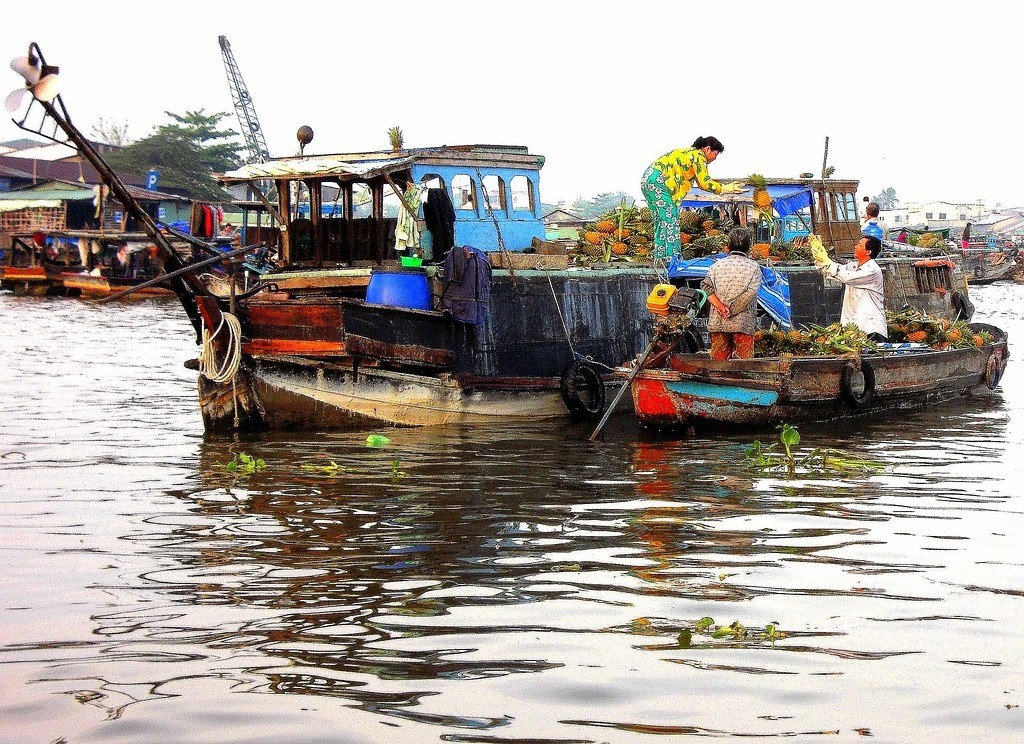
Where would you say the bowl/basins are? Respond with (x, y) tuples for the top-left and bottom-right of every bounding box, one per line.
(400, 256), (423, 267)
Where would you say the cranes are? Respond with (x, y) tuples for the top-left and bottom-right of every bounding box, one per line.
(219, 36), (271, 163)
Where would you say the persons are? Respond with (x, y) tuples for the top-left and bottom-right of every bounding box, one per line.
(701, 228), (761, 360)
(962, 223), (973, 249)
(948, 237), (958, 250)
(897, 227), (908, 243)
(924, 226), (929, 231)
(858, 196), (890, 241)
(217, 224), (234, 237)
(808, 233), (888, 343)
(641, 136), (750, 268)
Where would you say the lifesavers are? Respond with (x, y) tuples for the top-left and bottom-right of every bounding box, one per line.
(559, 357), (606, 421)
(974, 266), (986, 278)
(985, 352), (1003, 390)
(838, 357), (876, 411)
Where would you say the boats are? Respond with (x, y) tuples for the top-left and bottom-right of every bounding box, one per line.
(1, 227), (241, 301)
(884, 235), (1024, 284)
(615, 283), (1007, 423)
(4, 42), (975, 435)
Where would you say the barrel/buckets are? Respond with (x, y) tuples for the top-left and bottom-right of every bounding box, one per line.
(365, 266), (431, 310)
(200, 238), (232, 271)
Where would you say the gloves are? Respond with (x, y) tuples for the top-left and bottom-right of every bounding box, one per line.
(811, 247), (830, 266)
(720, 180), (750, 195)
(808, 233), (827, 255)
